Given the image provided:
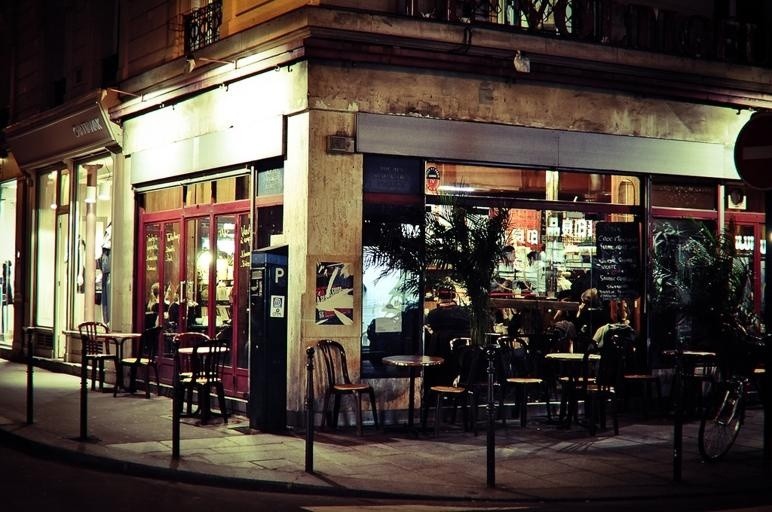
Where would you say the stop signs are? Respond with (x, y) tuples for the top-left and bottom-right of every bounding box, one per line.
(734, 113), (772, 191)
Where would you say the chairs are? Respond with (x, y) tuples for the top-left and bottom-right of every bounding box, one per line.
(605, 327), (666, 421)
(559, 335), (618, 438)
(318, 340), (380, 438)
(77, 322), (229, 423)
(430, 336), (479, 437)
(498, 336), (551, 427)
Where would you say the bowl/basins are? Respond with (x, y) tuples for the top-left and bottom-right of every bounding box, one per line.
(195, 317), (203, 324)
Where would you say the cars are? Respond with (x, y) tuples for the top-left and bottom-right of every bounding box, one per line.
(367, 302), (419, 371)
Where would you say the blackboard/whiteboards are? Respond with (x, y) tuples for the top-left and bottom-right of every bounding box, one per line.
(595, 222), (641, 301)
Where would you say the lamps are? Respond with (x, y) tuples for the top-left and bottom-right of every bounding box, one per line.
(513, 49), (531, 74)
(97, 85), (144, 102)
(184, 56), (238, 74)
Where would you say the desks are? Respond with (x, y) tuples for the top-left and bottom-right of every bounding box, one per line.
(383, 353), (444, 434)
(485, 297), (582, 314)
(663, 348), (718, 420)
(543, 352), (602, 428)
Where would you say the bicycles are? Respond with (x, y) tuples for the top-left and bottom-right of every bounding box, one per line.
(698, 321), (772, 464)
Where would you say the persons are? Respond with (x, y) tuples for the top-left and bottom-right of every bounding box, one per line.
(492, 246), (519, 296)
(168, 282), (201, 328)
(221, 288), (234, 340)
(526, 251), (573, 297)
(584, 297), (632, 356)
(426, 278), (469, 351)
(148, 282), (171, 315)
(573, 287), (611, 345)
